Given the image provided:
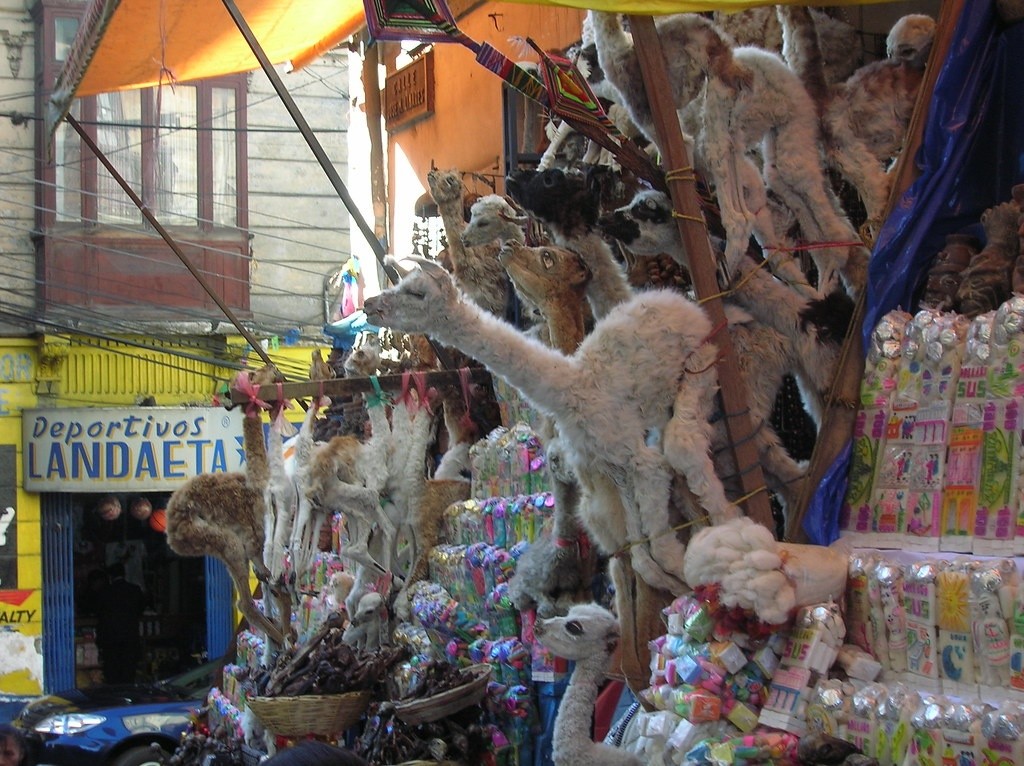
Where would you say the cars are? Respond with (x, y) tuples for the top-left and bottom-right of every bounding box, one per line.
(10, 657), (232, 766)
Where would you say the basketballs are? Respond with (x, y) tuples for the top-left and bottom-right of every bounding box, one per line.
(149, 508), (168, 533)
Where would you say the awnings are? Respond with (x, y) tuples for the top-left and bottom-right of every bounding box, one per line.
(44, 0), (464, 415)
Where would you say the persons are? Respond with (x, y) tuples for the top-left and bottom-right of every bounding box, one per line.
(94, 561), (142, 685)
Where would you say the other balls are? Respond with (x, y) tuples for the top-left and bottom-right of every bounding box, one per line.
(129, 497), (152, 520)
(99, 495), (121, 520)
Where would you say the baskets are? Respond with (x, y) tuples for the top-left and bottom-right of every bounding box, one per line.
(391, 663), (492, 725)
(246, 689), (370, 736)
